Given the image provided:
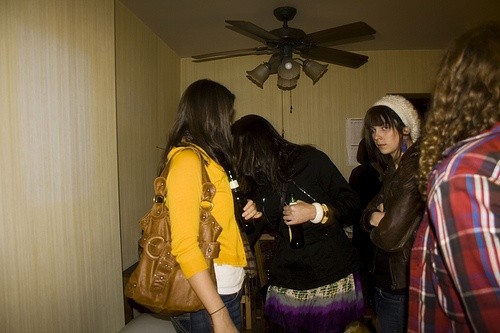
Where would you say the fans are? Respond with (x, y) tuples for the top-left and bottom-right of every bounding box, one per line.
(192, 7), (375, 68)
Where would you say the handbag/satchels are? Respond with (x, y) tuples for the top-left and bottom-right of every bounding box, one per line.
(265, 272), (365, 333)
(123, 145), (223, 312)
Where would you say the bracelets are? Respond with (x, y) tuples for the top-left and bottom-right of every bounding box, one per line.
(210, 305), (226, 316)
(311, 202), (323, 223)
(321, 203), (329, 224)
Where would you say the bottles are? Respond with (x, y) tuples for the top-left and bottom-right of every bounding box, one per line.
(227, 170), (255, 230)
(286, 192), (305, 248)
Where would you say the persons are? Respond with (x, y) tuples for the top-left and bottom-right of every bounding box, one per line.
(158, 79), (248, 333)
(408, 24), (500, 333)
(362, 94), (427, 333)
(230, 115), (361, 333)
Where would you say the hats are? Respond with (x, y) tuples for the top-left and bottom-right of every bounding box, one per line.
(374, 95), (420, 143)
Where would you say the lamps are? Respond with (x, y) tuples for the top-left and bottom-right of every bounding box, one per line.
(246, 45), (328, 139)
